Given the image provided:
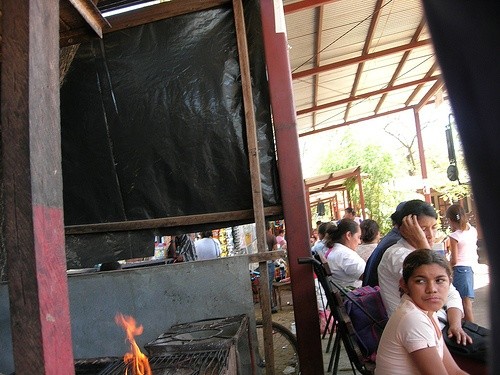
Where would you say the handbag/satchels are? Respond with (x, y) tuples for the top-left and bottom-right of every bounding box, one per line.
(438, 316), (490, 375)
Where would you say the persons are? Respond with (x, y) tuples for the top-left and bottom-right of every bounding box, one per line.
(375, 248), (472, 375)
(100, 261), (122, 271)
(165, 205), (478, 323)
(377, 199), (491, 375)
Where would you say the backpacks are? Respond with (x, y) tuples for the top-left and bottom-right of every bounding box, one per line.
(343, 285), (388, 361)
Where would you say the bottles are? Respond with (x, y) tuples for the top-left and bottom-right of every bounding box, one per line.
(275, 262), (285, 282)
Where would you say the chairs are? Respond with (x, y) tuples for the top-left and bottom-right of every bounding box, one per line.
(273, 279), (296, 311)
(297, 251), (376, 375)
